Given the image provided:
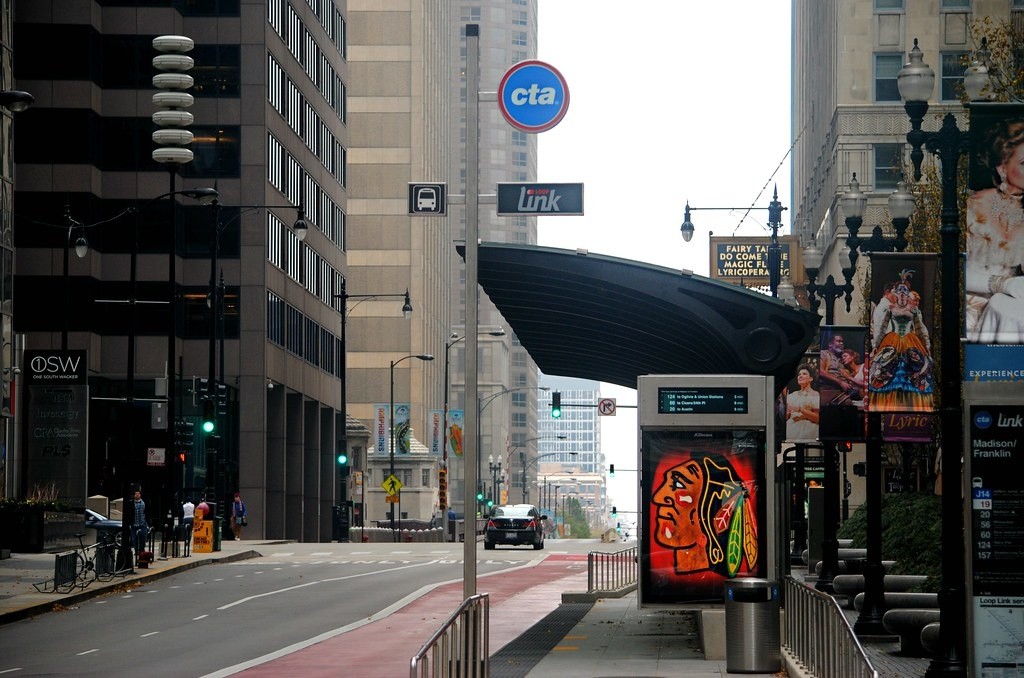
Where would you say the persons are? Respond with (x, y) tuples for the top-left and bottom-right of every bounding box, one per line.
(873, 281), (894, 352)
(804, 478), (822, 518)
(786, 364), (820, 440)
(183, 496), (196, 546)
(230, 493), (247, 541)
(908, 290), (922, 337)
(130, 490), (148, 556)
(868, 268), (934, 412)
(820, 333), (865, 408)
(839, 348), (865, 406)
(448, 507), (456, 520)
(967, 115), (1024, 344)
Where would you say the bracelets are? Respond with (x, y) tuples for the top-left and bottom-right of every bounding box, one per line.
(989, 274), (1008, 293)
(799, 406), (801, 411)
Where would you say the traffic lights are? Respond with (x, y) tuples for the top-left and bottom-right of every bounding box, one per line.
(552, 392), (560, 418)
(610, 463), (615, 477)
(204, 421), (214, 433)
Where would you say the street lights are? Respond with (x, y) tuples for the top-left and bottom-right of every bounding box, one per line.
(328, 286), (413, 544)
(472, 383), (599, 538)
(55, 183), (221, 351)
(441, 328), (507, 539)
(387, 350), (434, 530)
(204, 197), (311, 517)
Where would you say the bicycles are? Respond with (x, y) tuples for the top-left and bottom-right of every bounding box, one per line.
(66, 530), (128, 577)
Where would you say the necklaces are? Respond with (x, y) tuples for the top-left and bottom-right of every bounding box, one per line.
(990, 185), (1024, 234)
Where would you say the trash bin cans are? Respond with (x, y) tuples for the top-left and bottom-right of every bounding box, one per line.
(93, 518), (123, 572)
(723, 575), (782, 675)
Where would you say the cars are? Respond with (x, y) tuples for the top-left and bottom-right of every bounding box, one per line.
(484, 503), (548, 550)
(86, 508), (125, 547)
